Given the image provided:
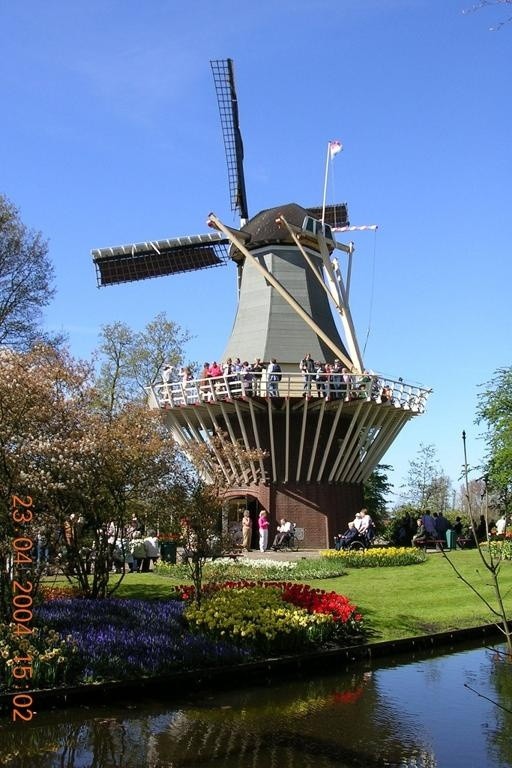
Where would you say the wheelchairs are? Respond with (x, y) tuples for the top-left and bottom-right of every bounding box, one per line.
(334, 529), (365, 549)
(270, 523), (299, 552)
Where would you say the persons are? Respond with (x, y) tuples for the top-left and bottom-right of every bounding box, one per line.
(331, 508), (511, 551)
(258, 509), (270, 553)
(230, 527), (243, 544)
(242, 509), (254, 552)
(14, 511), (160, 574)
(160, 353), (395, 409)
(203, 527), (221, 556)
(180, 527), (201, 566)
(270, 518), (294, 551)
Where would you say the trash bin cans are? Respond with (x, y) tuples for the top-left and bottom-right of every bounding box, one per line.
(446, 529), (457, 552)
(162, 542), (176, 563)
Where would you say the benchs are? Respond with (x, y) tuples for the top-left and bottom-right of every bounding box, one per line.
(134, 556), (158, 572)
(416, 538), (471, 549)
(183, 553), (243, 562)
